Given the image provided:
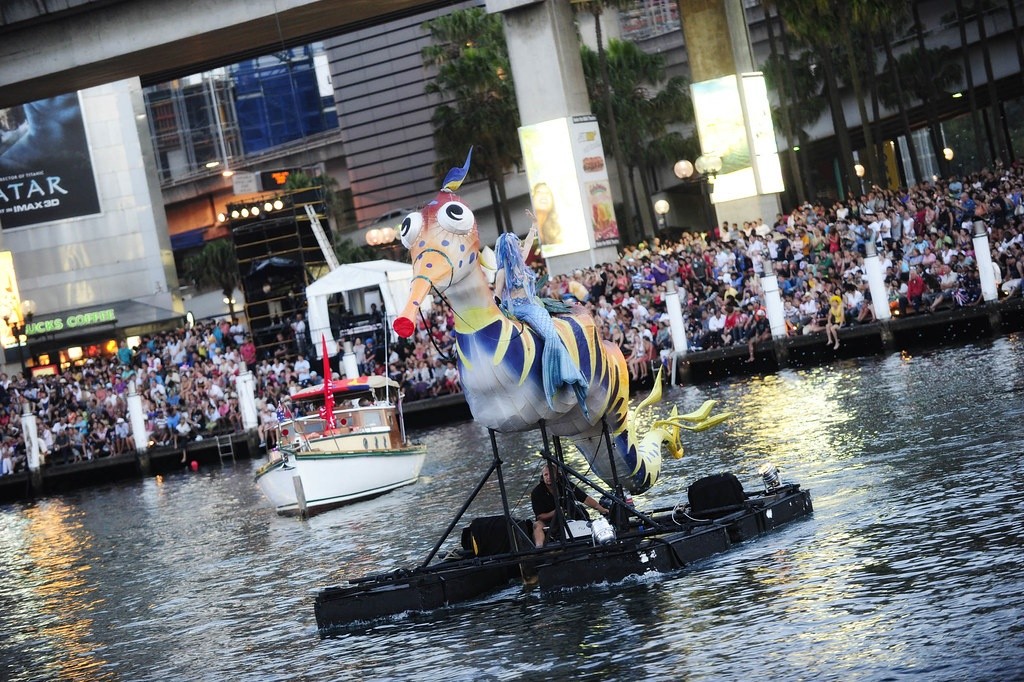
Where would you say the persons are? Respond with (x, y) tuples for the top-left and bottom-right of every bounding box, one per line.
(0, 157), (1024, 479)
(531, 465), (609, 549)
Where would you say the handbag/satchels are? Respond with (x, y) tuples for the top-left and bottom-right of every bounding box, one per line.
(564, 519), (592, 539)
(461, 515), (534, 556)
(686, 473), (749, 512)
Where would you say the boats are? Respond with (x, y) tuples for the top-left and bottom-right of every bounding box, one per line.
(313, 142), (814, 638)
(253, 311), (428, 519)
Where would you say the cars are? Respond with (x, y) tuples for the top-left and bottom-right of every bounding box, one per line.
(371, 208), (416, 224)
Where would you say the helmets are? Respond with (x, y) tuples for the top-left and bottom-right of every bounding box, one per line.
(599, 488), (635, 517)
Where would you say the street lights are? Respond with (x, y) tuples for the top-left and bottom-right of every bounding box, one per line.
(223, 168), (273, 258)
(673, 153), (724, 240)
(364, 225), (404, 260)
(0, 300), (37, 412)
(652, 197), (671, 230)
